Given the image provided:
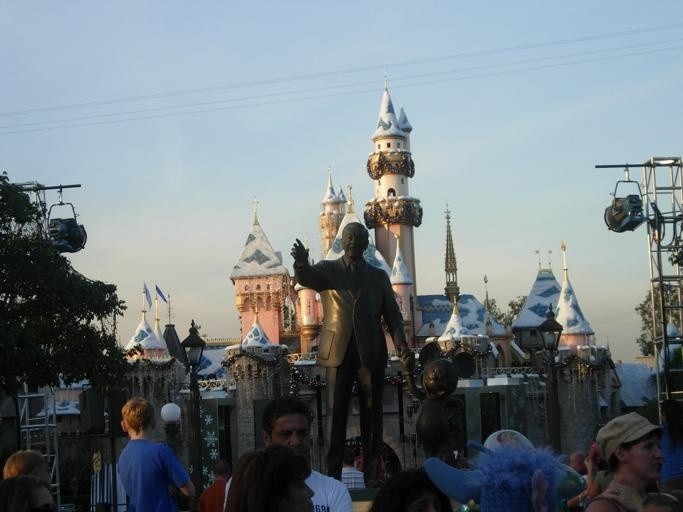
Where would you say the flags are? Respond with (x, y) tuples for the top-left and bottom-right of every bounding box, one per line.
(144, 283), (152, 309)
(156, 286), (168, 303)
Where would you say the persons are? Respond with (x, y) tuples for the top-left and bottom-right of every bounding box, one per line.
(223, 396), (355, 511)
(116, 396), (197, 511)
(370, 465), (453, 512)
(290, 221), (413, 487)
(0, 477), (59, 511)
(222, 444), (317, 512)
(3, 448), (48, 483)
(562, 399), (683, 511)
(197, 459), (226, 511)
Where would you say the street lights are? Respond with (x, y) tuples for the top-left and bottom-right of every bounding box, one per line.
(536, 301), (564, 457)
(160, 403), (182, 459)
(180, 319), (207, 511)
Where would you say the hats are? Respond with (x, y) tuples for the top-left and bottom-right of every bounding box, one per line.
(597, 411), (664, 463)
